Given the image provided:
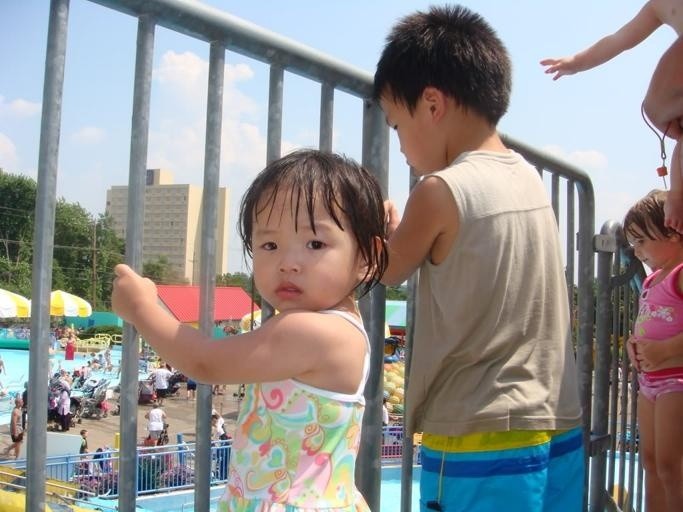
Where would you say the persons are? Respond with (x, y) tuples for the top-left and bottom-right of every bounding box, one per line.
(367, 5), (588, 512)
(111, 148), (388, 512)
(0, 322), (247, 502)
(539, 0), (683, 512)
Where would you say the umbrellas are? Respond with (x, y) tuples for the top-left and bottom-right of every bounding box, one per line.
(50, 290), (92, 317)
(0, 289), (31, 317)
